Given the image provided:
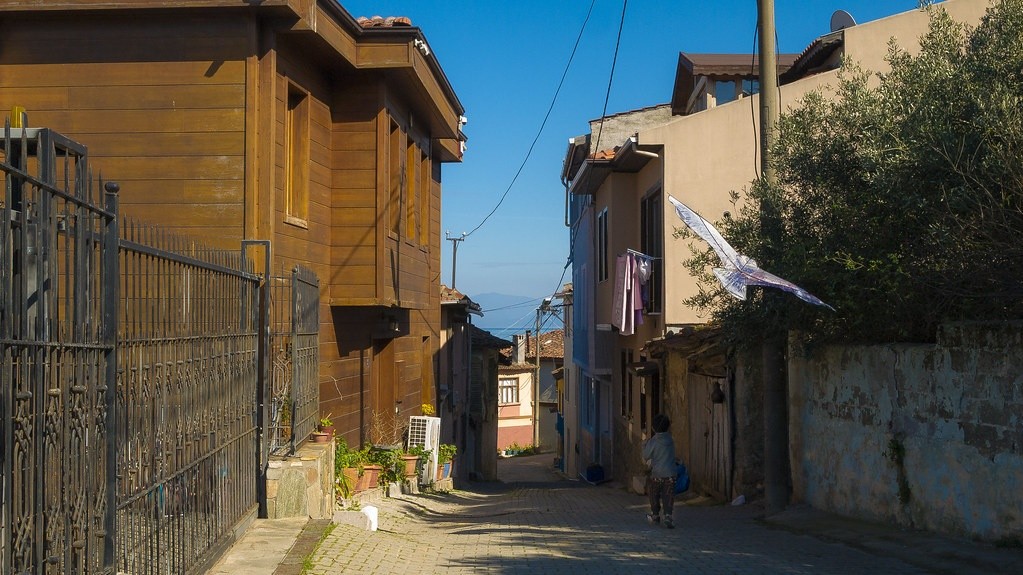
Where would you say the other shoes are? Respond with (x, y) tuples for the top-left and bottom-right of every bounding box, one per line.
(646, 513), (660, 523)
(662, 516), (676, 529)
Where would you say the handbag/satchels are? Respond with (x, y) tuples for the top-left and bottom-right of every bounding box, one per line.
(673, 462), (690, 495)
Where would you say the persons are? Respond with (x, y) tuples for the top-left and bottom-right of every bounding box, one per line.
(642, 414), (677, 530)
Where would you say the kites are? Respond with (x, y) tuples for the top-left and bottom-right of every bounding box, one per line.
(668, 195), (836, 312)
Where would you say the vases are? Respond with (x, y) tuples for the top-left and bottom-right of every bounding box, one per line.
(423, 414), (428, 416)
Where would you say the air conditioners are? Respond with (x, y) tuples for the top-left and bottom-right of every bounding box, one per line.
(408, 416), (441, 484)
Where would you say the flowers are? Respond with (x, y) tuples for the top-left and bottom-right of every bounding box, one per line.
(421, 403), (434, 416)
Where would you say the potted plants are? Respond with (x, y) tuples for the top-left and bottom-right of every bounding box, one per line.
(312, 418), (457, 496)
(501, 441), (544, 455)
